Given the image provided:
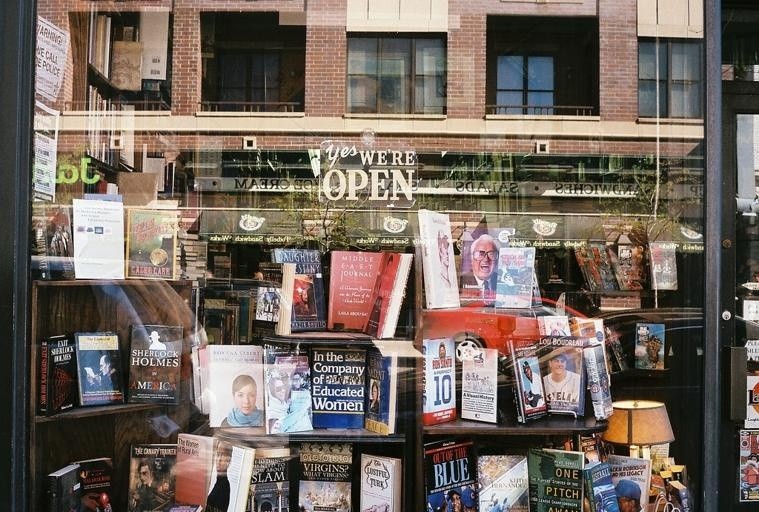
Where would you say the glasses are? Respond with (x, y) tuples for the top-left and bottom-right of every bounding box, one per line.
(473, 250), (499, 261)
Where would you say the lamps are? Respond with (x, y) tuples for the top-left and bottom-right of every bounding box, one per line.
(598, 396), (678, 492)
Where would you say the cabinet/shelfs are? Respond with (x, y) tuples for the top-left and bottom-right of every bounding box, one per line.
(27, 275), (198, 512)
(418, 229), (611, 512)
(199, 237), (418, 512)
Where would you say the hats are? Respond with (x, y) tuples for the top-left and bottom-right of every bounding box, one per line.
(615, 480), (641, 501)
(523, 361), (530, 373)
(448, 487), (462, 495)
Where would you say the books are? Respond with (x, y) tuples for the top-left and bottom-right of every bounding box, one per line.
(31, 169), (758, 512)
(87, 10), (168, 168)
